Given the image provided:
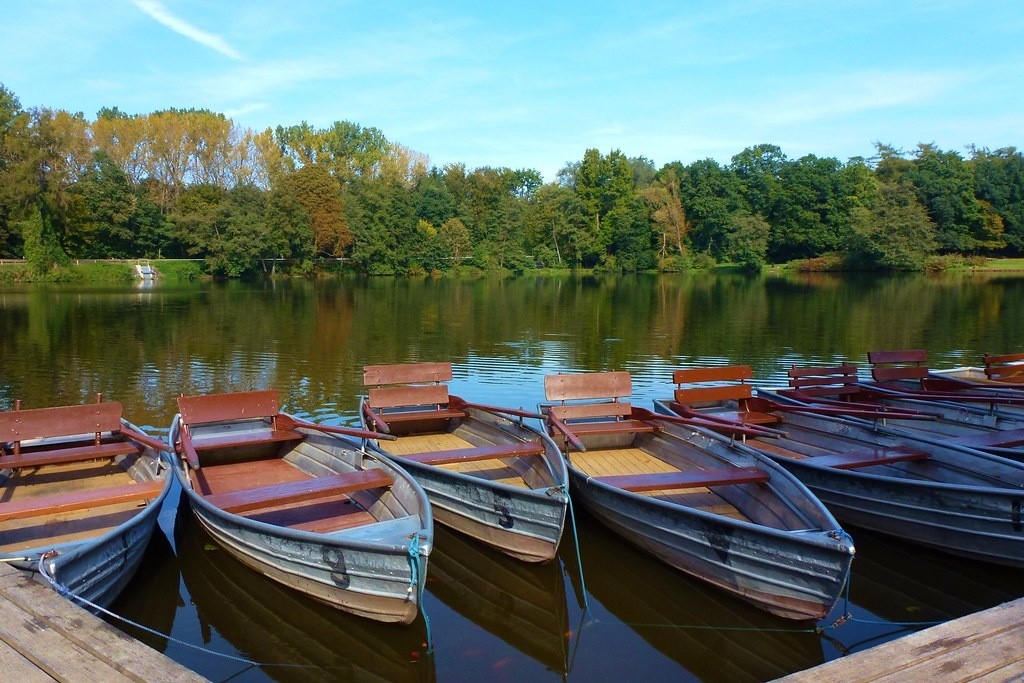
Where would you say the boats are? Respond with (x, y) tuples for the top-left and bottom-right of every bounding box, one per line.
(848, 351), (1024, 444)
(754, 364), (1024, 459)
(651, 367), (1024, 569)
(165, 388), (435, 626)
(171, 503), (438, 683)
(0, 392), (176, 622)
(532, 370), (857, 626)
(840, 518), (1024, 626)
(572, 501), (825, 683)
(358, 363), (571, 565)
(424, 517), (573, 683)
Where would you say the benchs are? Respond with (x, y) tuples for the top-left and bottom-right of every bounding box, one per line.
(983, 352), (1024, 383)
(175, 389), (307, 452)
(363, 362), (470, 423)
(672, 366), (785, 430)
(0, 393), (146, 469)
(788, 361), (860, 401)
(544, 369), (665, 437)
(868, 350), (930, 391)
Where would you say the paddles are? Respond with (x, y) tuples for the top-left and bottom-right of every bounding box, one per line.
(446, 395), (549, 420)
(669, 402), (791, 439)
(363, 401), (391, 433)
(623, 406), (781, 440)
(738, 397), (938, 421)
(838, 379), (1024, 403)
(272, 414), (397, 442)
(548, 410), (587, 453)
(117, 427), (176, 454)
(180, 427), (199, 470)
(776, 390), (944, 419)
